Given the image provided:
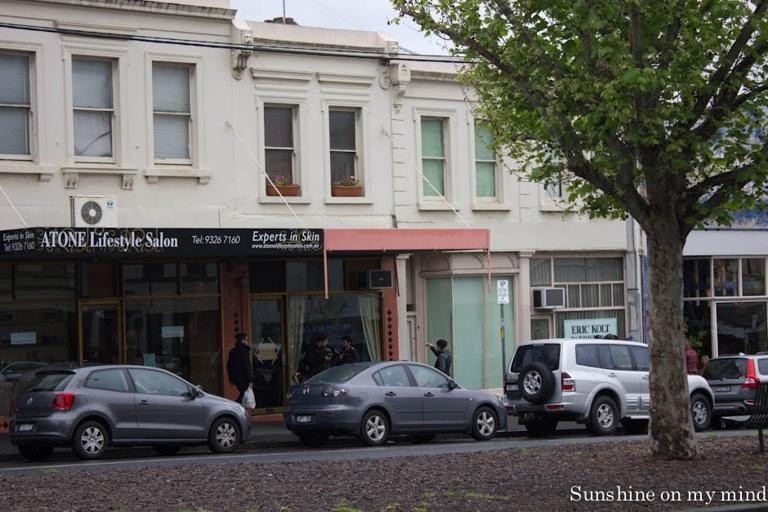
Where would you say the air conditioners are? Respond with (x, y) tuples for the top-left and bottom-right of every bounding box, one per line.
(531, 286), (567, 310)
(68, 194), (121, 229)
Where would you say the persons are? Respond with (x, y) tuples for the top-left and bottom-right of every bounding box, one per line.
(425, 340), (452, 375)
(227, 332), (255, 404)
(683, 339), (698, 375)
(301, 333), (333, 382)
(699, 354), (710, 375)
(336, 336), (360, 365)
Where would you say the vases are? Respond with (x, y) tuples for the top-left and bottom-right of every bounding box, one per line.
(332, 186), (363, 196)
(267, 184), (301, 196)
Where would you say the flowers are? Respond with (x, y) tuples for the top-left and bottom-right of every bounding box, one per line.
(271, 174), (290, 185)
(333, 175), (361, 185)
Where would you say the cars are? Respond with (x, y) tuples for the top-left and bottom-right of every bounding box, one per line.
(283, 362), (508, 447)
(9, 363), (249, 455)
(138, 356), (357, 407)
(0, 359), (51, 417)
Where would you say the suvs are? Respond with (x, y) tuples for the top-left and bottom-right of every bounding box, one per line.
(502, 337), (714, 436)
(698, 350), (767, 429)
